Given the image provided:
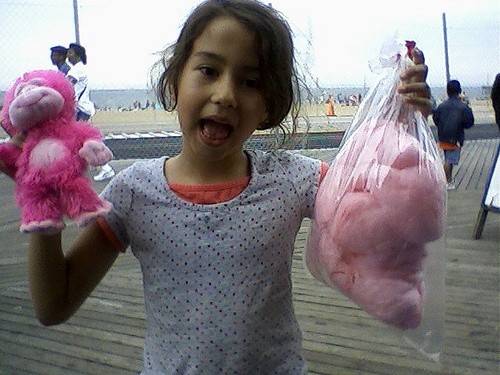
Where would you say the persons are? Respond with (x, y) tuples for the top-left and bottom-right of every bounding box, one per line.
(433, 80), (475, 192)
(50, 43), (115, 182)
(11, 0), (434, 375)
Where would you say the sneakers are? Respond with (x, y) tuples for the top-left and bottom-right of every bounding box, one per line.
(94, 167), (116, 182)
(447, 181), (455, 191)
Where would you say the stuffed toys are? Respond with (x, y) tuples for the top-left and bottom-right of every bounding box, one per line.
(306, 123), (445, 331)
(0, 70), (114, 233)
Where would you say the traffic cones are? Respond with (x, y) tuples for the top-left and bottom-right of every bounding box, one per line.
(326, 100), (336, 116)
